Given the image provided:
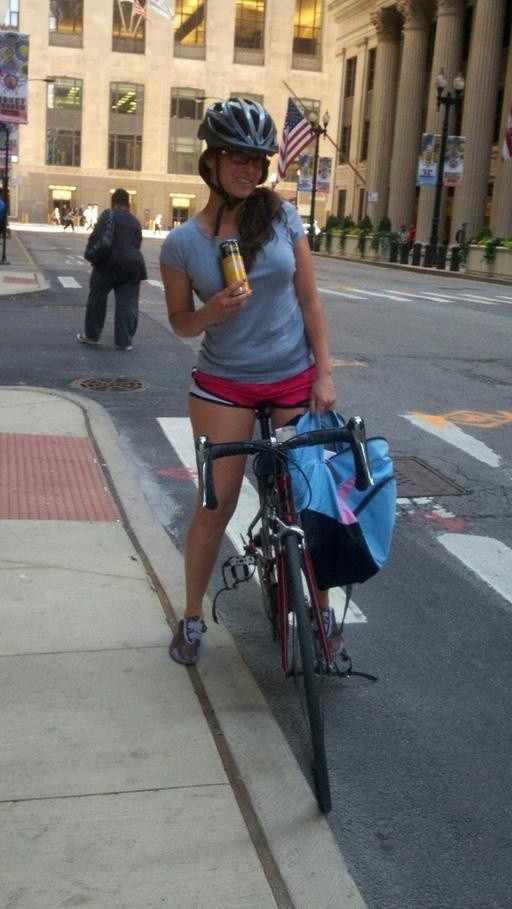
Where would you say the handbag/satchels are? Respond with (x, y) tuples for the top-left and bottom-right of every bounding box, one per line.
(82, 214), (118, 266)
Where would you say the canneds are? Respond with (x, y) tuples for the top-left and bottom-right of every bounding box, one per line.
(219, 239), (249, 296)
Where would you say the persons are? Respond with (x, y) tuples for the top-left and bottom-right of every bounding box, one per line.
(398, 225), (407, 244)
(76, 189), (148, 351)
(170, 98), (336, 665)
(152, 212), (164, 232)
(49, 203), (98, 230)
(408, 222), (415, 246)
(456, 222), (468, 246)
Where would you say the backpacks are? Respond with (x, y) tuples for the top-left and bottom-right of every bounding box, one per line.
(286, 402), (398, 594)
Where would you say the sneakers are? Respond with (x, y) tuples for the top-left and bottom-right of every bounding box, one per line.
(76, 333), (101, 347)
(113, 342), (135, 352)
(168, 611), (208, 665)
(311, 602), (347, 663)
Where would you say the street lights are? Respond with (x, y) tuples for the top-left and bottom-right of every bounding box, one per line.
(2, 77), (56, 264)
(425, 67), (466, 266)
(305, 107), (331, 251)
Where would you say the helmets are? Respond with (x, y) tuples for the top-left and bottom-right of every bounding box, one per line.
(194, 95), (283, 155)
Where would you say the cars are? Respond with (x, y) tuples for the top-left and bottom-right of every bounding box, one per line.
(300, 224), (321, 236)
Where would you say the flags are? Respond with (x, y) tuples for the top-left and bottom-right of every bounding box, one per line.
(132, 0), (146, 19)
(147, 1), (176, 20)
(502, 105), (511, 160)
(276, 98), (316, 184)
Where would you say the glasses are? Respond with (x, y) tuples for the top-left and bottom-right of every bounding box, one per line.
(206, 146), (272, 171)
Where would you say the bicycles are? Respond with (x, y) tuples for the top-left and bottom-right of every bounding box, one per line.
(195, 393), (372, 813)
